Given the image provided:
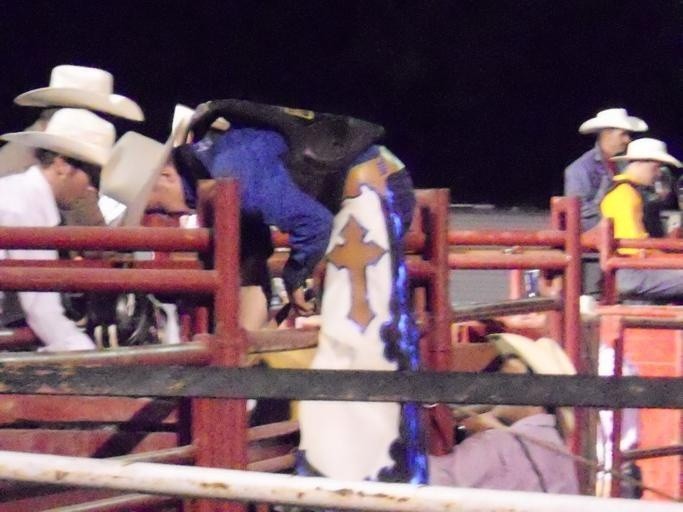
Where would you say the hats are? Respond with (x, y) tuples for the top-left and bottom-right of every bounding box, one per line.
(14, 62), (147, 122)
(608, 137), (682, 169)
(486, 332), (579, 435)
(1, 107), (118, 166)
(578, 107), (649, 134)
(98, 100), (198, 227)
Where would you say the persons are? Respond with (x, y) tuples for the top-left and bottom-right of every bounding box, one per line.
(0, 65), (112, 255)
(427, 334), (579, 495)
(597, 139), (682, 302)
(0, 109), (115, 354)
(97, 124), (414, 373)
(535, 247), (643, 501)
(563, 109), (648, 292)
